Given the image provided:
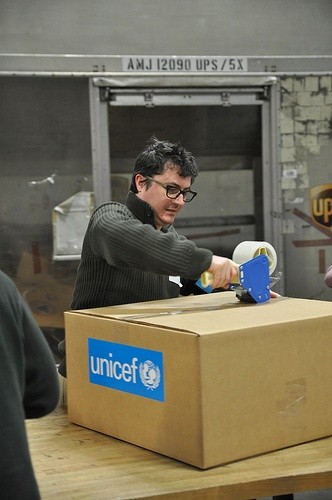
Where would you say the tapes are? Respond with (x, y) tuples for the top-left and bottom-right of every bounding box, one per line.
(232, 240), (278, 276)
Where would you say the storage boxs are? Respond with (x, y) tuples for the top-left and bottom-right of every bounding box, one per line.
(18, 278), (74, 329)
(64, 289), (332, 470)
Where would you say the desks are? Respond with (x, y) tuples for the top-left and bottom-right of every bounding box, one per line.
(24, 405), (332, 500)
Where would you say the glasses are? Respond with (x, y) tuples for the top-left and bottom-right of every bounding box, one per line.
(145, 174), (198, 203)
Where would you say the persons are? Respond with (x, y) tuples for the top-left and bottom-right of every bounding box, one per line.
(57, 136), (281, 405)
(0, 268), (61, 500)
